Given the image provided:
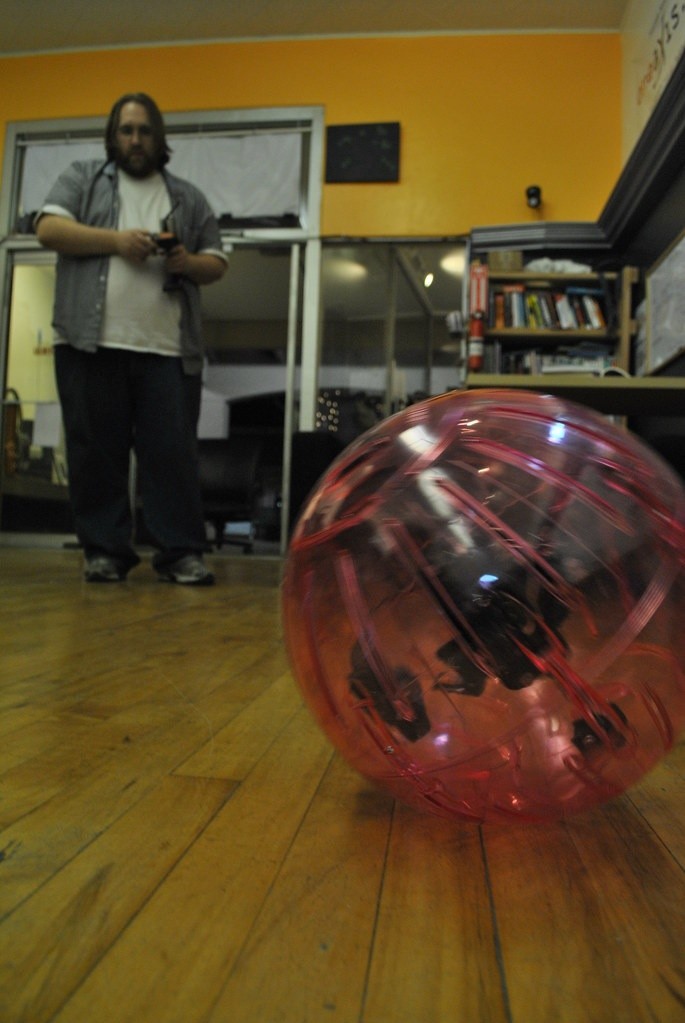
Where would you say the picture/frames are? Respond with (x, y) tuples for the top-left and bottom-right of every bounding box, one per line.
(645, 230), (685, 375)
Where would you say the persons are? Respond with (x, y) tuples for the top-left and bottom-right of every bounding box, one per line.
(32, 92), (230, 583)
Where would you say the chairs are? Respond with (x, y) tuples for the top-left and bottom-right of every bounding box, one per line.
(196, 439), (255, 555)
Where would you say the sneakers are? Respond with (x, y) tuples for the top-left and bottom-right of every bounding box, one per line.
(84, 556), (124, 581)
(154, 553), (215, 587)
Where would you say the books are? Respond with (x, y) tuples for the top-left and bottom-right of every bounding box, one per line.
(501, 350), (612, 375)
(542, 365), (629, 377)
(494, 291), (604, 329)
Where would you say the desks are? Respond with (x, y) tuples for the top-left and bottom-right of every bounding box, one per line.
(460, 372), (685, 417)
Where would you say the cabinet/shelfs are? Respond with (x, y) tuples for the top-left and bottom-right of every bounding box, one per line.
(468, 250), (639, 433)
